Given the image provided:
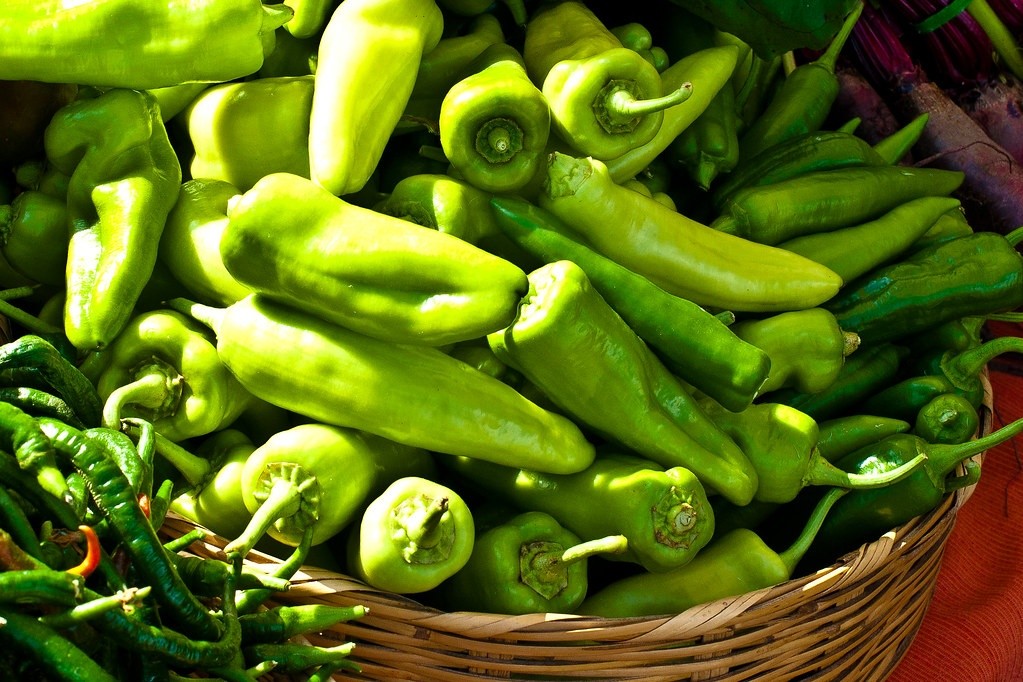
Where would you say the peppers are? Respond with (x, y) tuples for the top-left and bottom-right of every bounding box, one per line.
(0, 0), (1023, 682)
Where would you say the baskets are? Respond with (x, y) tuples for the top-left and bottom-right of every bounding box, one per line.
(100, 360), (993, 682)
